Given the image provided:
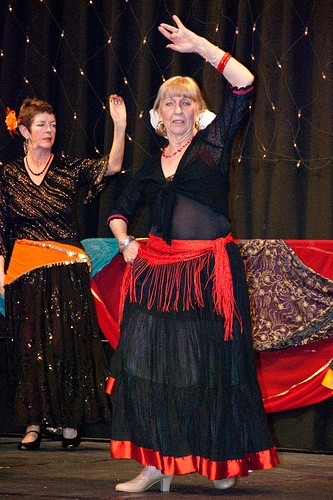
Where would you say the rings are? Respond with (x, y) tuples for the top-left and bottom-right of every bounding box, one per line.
(176, 28), (179, 32)
(115, 102), (117, 104)
(118, 102), (121, 104)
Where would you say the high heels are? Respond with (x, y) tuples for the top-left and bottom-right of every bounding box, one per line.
(18, 430), (43, 451)
(116, 468), (172, 492)
(62, 429), (81, 451)
(214, 479), (234, 489)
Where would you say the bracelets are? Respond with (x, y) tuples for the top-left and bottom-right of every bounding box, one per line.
(217, 52), (232, 74)
(209, 46), (218, 67)
(118, 235), (135, 253)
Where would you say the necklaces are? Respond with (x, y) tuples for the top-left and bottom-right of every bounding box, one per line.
(26, 153), (52, 176)
(161, 135), (195, 158)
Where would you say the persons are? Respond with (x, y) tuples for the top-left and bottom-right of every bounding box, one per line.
(105, 14), (280, 494)
(0, 94), (126, 451)
